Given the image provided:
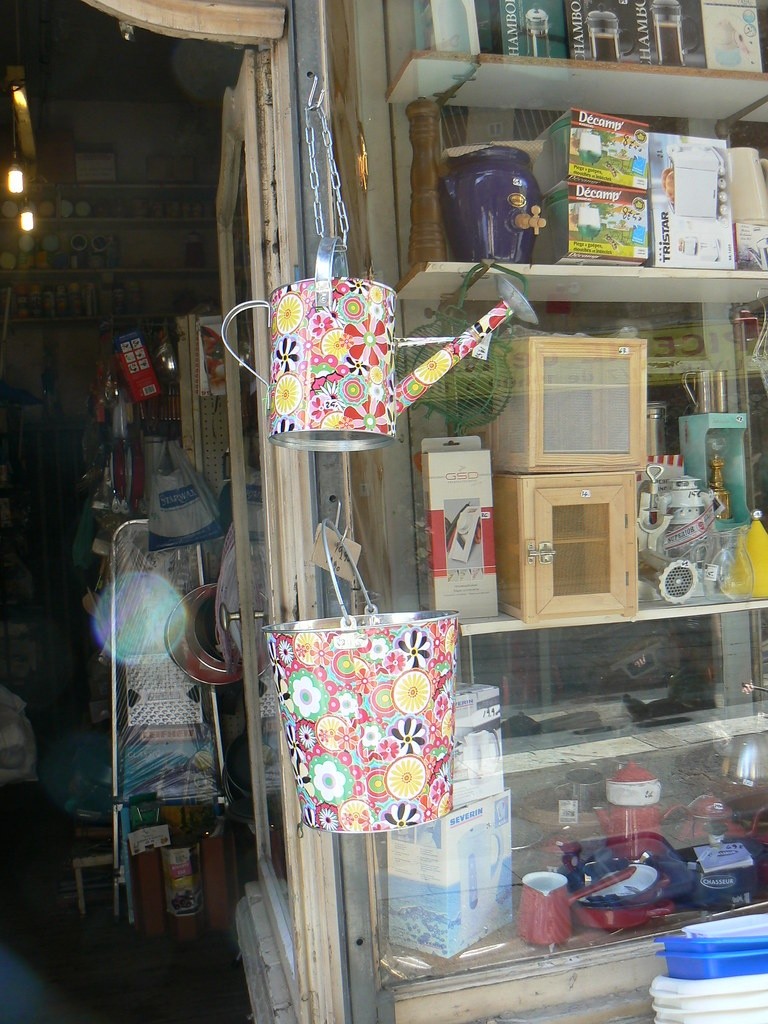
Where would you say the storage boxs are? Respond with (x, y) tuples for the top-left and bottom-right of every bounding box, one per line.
(386, 787), (514, 960)
(111, 329), (163, 403)
(530, 107), (650, 192)
(534, 180), (649, 266)
(732, 224), (767, 271)
(421, 432), (499, 618)
(419, 1), (763, 73)
(646, 133), (736, 270)
(450, 680), (507, 808)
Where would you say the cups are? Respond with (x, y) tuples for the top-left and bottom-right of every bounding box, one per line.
(682, 370), (728, 414)
(646, 402), (667, 458)
(553, 768), (604, 813)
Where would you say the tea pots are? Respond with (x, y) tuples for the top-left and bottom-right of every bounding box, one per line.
(592, 761), (694, 862)
(663, 792), (768, 845)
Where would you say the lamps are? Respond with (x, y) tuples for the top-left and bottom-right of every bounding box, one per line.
(6, 84), (25, 193)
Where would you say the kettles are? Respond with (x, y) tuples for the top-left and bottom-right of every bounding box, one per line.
(458, 824), (504, 945)
(464, 727), (499, 781)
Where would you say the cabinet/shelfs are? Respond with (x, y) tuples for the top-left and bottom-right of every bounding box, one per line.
(0, 179), (247, 324)
(384, 49), (768, 637)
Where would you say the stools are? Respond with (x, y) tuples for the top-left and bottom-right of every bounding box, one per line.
(72, 839), (114, 918)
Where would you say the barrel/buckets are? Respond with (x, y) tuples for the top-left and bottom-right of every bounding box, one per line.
(259, 519), (459, 834)
(655, 950), (768, 980)
(654, 937), (768, 953)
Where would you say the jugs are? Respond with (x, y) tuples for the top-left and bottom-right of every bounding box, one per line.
(516, 866), (635, 946)
(691, 525), (754, 602)
(221, 238), (537, 452)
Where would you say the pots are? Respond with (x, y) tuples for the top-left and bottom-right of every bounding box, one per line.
(571, 889), (675, 930)
(565, 863), (671, 906)
